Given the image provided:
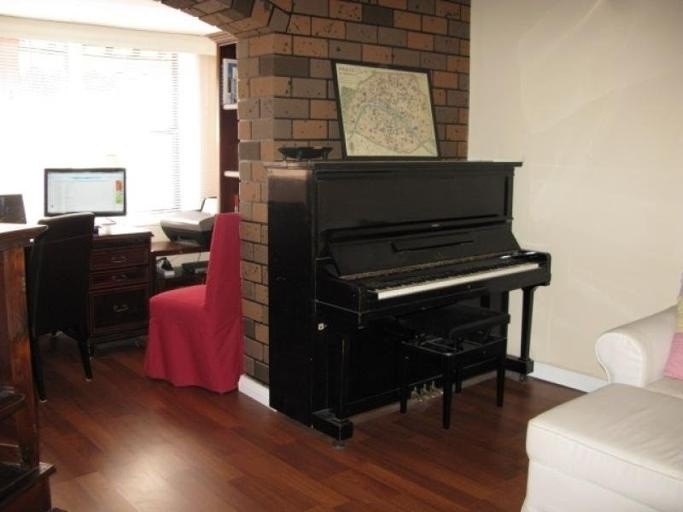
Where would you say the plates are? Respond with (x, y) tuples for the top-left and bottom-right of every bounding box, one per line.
(0, 221), (61, 512)
(216, 40), (239, 211)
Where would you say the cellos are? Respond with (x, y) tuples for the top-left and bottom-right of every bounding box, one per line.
(161, 196), (218, 248)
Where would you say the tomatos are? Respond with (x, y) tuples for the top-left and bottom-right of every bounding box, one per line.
(267, 156), (551, 442)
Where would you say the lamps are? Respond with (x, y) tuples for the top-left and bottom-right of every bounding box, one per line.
(31, 212), (239, 404)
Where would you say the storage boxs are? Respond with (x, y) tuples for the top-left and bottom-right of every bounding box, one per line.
(514, 383), (683, 512)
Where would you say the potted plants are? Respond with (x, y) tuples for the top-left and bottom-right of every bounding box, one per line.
(330, 59), (442, 159)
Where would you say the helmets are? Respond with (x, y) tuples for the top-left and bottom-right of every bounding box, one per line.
(276, 146), (332, 160)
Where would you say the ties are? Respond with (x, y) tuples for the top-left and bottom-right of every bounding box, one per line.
(44, 168), (126, 223)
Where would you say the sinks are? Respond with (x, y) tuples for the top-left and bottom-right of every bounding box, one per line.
(592, 275), (683, 393)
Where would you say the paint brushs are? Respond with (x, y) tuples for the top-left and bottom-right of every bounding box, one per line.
(156, 259), (174, 277)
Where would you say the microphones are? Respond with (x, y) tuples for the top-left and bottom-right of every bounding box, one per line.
(399, 330), (506, 427)
